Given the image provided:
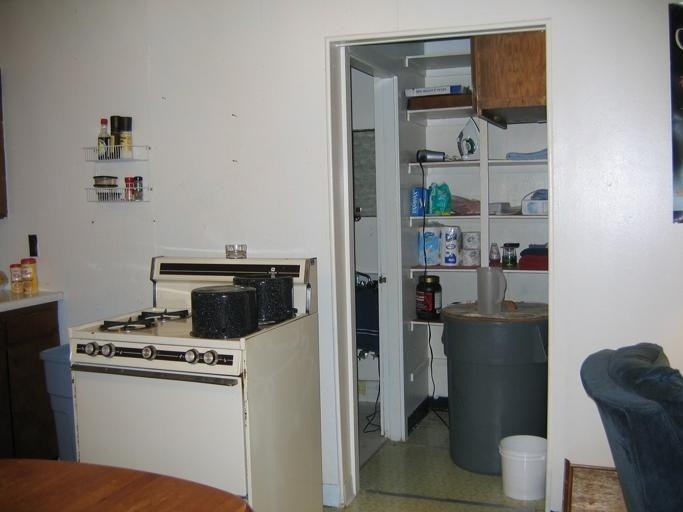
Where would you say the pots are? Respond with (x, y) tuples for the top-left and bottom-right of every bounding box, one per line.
(189, 284), (258, 343)
(232, 272), (297, 323)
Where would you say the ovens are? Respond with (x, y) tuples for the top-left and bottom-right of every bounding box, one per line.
(68, 363), (243, 499)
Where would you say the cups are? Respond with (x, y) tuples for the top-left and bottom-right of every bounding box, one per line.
(224, 243), (248, 260)
(475, 266), (507, 316)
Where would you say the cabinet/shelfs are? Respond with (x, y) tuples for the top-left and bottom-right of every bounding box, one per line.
(469, 33), (547, 134)
(83, 145), (151, 204)
(405, 55), (547, 328)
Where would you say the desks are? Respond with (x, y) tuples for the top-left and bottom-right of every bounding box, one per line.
(1, 287), (63, 458)
(1, 458), (253, 511)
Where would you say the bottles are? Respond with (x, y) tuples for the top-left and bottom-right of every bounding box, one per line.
(10, 263), (24, 295)
(499, 242), (521, 269)
(133, 176), (144, 201)
(489, 242), (500, 267)
(97, 117), (108, 161)
(124, 176), (135, 202)
(21, 257), (38, 297)
(414, 274), (443, 322)
(109, 116), (120, 160)
(118, 116), (133, 160)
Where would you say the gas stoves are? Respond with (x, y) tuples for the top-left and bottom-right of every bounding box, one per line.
(70, 306), (295, 342)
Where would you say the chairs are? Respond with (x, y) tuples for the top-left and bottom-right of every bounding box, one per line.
(581, 341), (683, 512)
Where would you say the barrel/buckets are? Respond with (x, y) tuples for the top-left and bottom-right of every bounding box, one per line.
(500, 435), (548, 502)
(441, 315), (548, 475)
